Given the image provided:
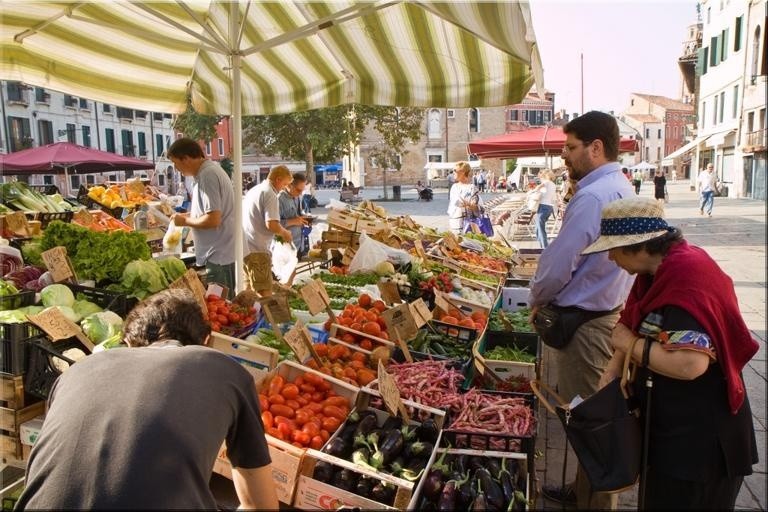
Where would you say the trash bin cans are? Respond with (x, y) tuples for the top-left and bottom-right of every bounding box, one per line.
(393, 185), (401, 201)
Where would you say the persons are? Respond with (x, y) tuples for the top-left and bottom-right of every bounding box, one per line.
(340, 183), (347, 202)
(160, 137), (236, 296)
(348, 180), (354, 187)
(239, 164), (293, 297)
(244, 176), (255, 190)
(526, 168), (558, 250)
(446, 171), (456, 200)
(11, 287), (282, 511)
(671, 167), (678, 184)
(652, 168), (667, 204)
(472, 170), (506, 192)
(694, 163), (720, 217)
(444, 160), (480, 238)
(277, 172), (318, 254)
(522, 106), (642, 511)
(414, 181), (430, 200)
(632, 168), (642, 196)
(578, 194), (760, 512)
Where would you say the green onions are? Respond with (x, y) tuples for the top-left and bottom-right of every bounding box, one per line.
(0, 182), (65, 220)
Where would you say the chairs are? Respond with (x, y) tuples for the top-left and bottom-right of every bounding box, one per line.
(481, 192), (568, 242)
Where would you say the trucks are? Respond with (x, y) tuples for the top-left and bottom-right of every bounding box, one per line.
(507, 156), (568, 190)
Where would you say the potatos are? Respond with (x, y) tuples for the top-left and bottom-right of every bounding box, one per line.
(258, 342), (390, 450)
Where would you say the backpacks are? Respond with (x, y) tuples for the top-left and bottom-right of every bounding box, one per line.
(534, 306), (611, 350)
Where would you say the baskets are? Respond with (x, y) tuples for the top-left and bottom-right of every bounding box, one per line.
(0, 284), (138, 402)
(392, 319), (535, 503)
(413, 253), (543, 298)
(3, 184), (140, 229)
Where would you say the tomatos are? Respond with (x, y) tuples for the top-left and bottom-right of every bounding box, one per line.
(325, 294), (391, 351)
(438, 304), (487, 339)
(203, 294), (257, 332)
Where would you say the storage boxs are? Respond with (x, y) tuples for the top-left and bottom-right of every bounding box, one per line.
(0, 370), (49, 468)
(200, 203), (550, 511)
(18, 413), (48, 465)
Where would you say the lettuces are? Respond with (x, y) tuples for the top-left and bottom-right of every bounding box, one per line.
(21, 220), (189, 301)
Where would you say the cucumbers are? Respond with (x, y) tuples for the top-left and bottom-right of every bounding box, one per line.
(408, 329), (474, 361)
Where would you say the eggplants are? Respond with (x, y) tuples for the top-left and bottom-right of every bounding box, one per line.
(313, 411), (525, 512)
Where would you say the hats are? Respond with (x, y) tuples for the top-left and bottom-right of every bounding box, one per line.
(575, 194), (671, 257)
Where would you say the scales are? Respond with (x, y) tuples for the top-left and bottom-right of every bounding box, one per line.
(514, 248), (545, 275)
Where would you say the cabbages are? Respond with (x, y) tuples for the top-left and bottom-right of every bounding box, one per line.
(0, 284), (124, 350)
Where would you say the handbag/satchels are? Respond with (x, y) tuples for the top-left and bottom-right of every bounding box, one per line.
(555, 379), (646, 499)
(526, 189), (543, 214)
(457, 202), (494, 239)
